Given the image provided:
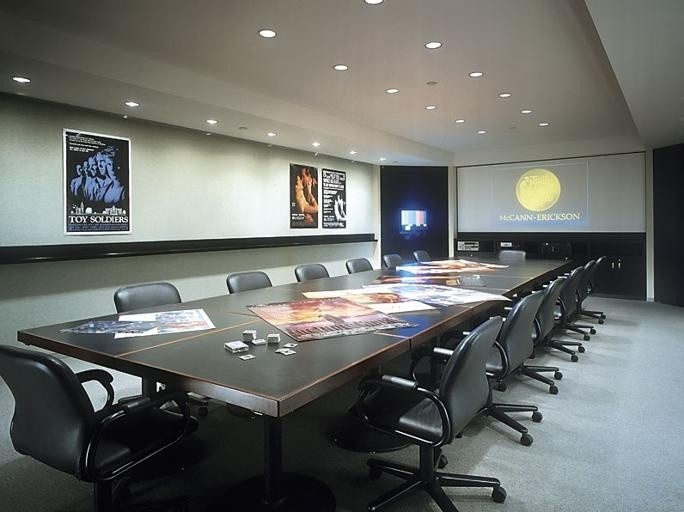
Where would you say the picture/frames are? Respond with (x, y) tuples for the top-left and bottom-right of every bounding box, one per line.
(322, 168), (347, 228)
(62, 128), (132, 236)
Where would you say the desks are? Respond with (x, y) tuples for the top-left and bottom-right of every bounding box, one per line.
(18, 259), (574, 511)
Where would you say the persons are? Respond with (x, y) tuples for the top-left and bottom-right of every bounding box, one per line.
(293, 167), (318, 226)
(317, 312), (346, 325)
(69, 147), (129, 213)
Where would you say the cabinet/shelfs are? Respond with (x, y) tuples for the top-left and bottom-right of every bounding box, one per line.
(454, 233), (646, 301)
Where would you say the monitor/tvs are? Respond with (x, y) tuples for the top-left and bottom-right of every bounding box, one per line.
(398, 207), (431, 236)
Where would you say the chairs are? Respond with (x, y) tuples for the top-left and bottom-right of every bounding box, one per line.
(576, 255), (611, 324)
(359, 316), (506, 512)
(228, 272), (271, 294)
(499, 251), (525, 262)
(410, 290), (545, 446)
(114, 283), (208, 415)
(413, 249), (431, 263)
(383, 254), (403, 267)
(543, 266), (585, 362)
(295, 264), (330, 282)
(0, 344), (199, 512)
(501, 277), (567, 396)
(563, 259), (596, 341)
(347, 257), (372, 274)
